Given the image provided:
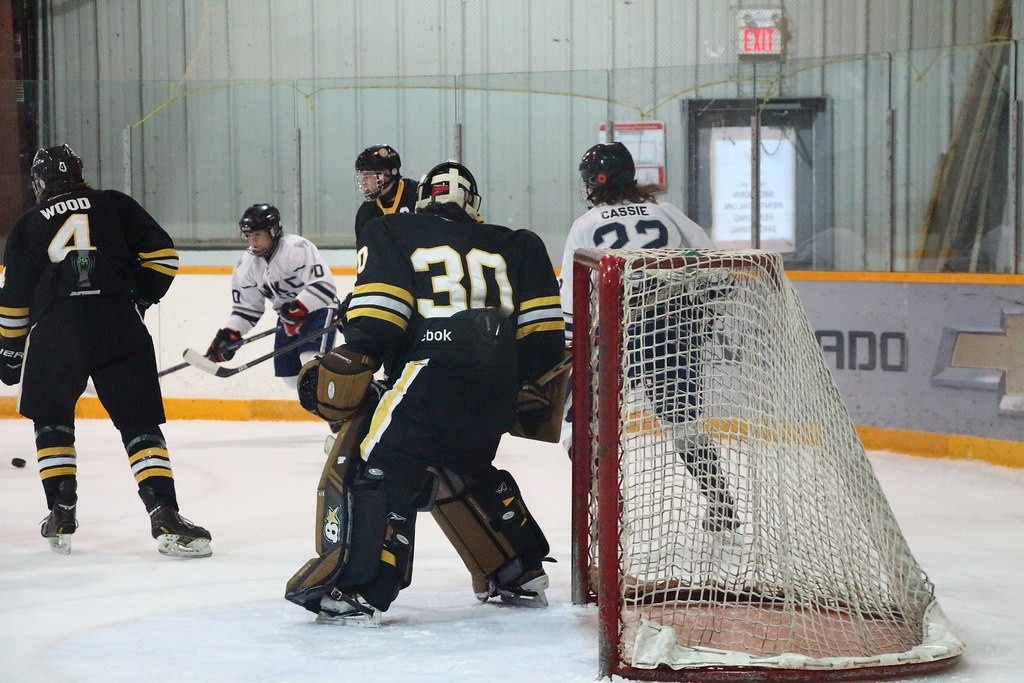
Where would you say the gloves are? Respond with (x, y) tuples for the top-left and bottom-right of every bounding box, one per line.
(0, 332), (26, 385)
(276, 299), (307, 336)
(207, 328), (244, 363)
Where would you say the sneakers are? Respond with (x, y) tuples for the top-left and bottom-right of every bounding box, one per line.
(138, 477), (213, 558)
(473, 474), (550, 609)
(317, 490), (411, 628)
(38, 479), (78, 555)
(678, 447), (742, 545)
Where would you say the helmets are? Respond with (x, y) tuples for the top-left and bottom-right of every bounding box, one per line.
(30, 144), (83, 200)
(415, 161), (482, 221)
(577, 140), (635, 202)
(355, 144), (402, 201)
(238, 203), (284, 258)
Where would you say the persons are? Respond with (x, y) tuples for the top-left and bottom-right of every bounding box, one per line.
(558, 143), (749, 545)
(284, 161), (573, 629)
(205, 204), (344, 439)
(0, 145), (215, 559)
(332, 145), (419, 380)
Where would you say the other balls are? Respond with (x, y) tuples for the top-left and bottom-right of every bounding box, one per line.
(12, 458), (26, 467)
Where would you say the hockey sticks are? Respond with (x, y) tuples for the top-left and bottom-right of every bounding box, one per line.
(183, 314), (342, 378)
(534, 285), (677, 386)
(85, 324), (283, 395)
